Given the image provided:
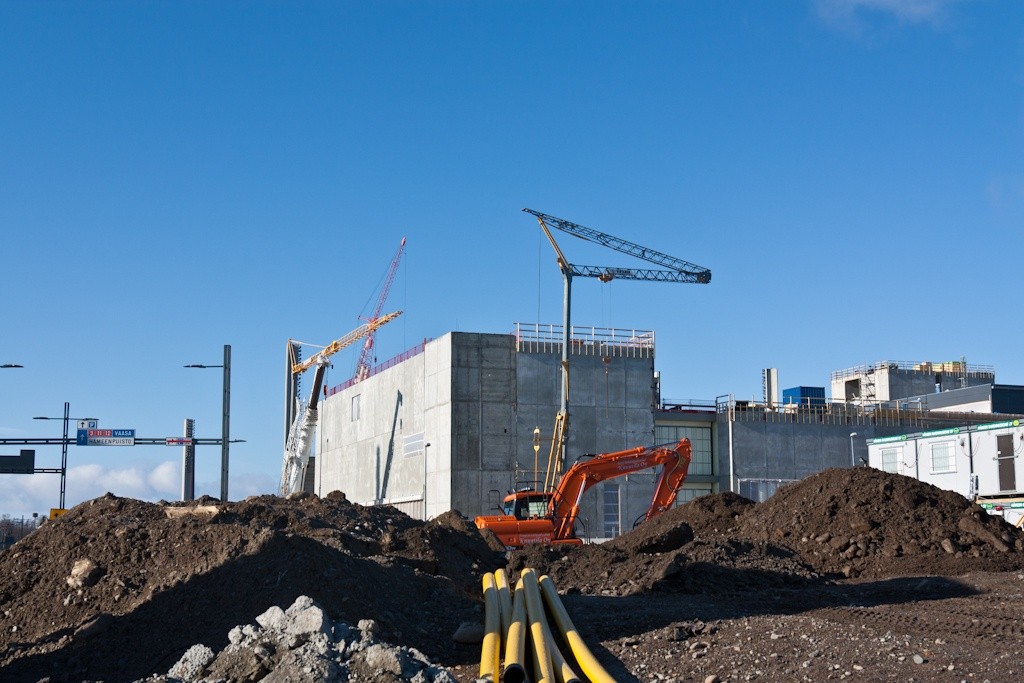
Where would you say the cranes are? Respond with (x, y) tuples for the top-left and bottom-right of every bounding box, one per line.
(353, 238), (407, 383)
(290, 311), (404, 376)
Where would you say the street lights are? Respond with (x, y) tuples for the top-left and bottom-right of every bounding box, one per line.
(183, 344), (233, 504)
(33, 401), (100, 509)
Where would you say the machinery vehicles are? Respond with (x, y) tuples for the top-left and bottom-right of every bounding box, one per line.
(474, 434), (692, 551)
